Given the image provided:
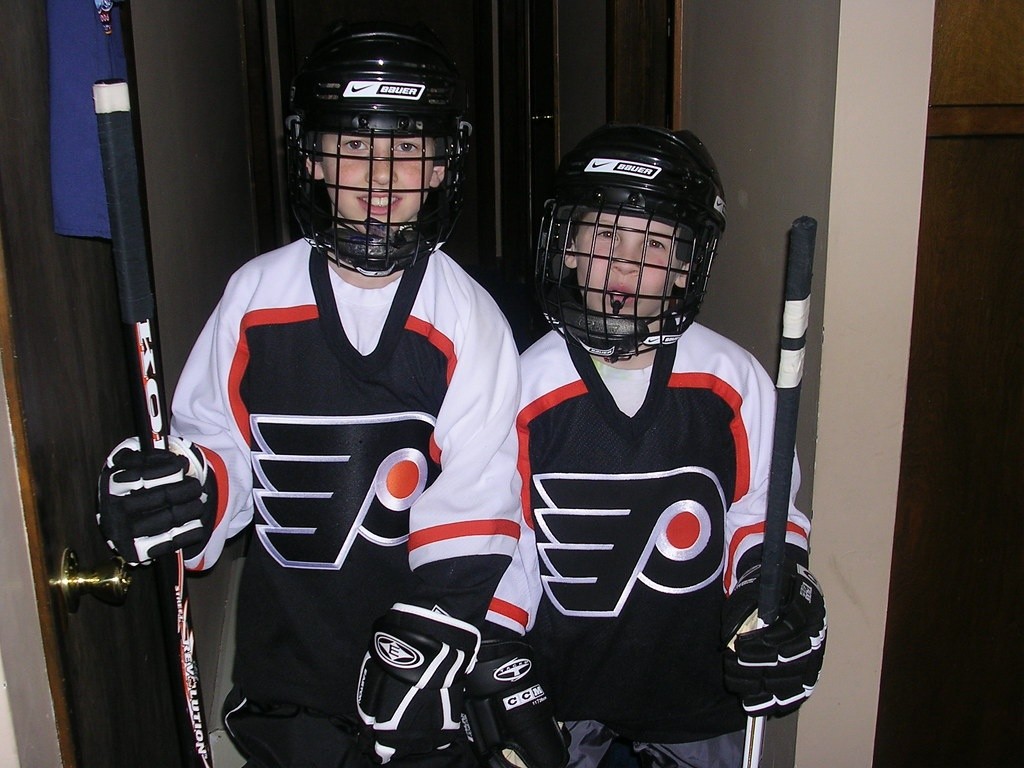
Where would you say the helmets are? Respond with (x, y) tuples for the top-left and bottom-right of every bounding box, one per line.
(534, 122), (729, 362)
(286, 19), (477, 277)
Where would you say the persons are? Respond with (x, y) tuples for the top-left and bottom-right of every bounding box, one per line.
(465, 122), (829, 768)
(96, 22), (523, 768)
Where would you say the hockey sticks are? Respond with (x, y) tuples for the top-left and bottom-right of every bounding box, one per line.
(735, 212), (818, 767)
(87, 82), (214, 768)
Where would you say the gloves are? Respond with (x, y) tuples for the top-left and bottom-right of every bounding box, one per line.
(95, 431), (218, 571)
(353, 596), (483, 768)
(465, 636), (572, 768)
(723, 539), (829, 715)
